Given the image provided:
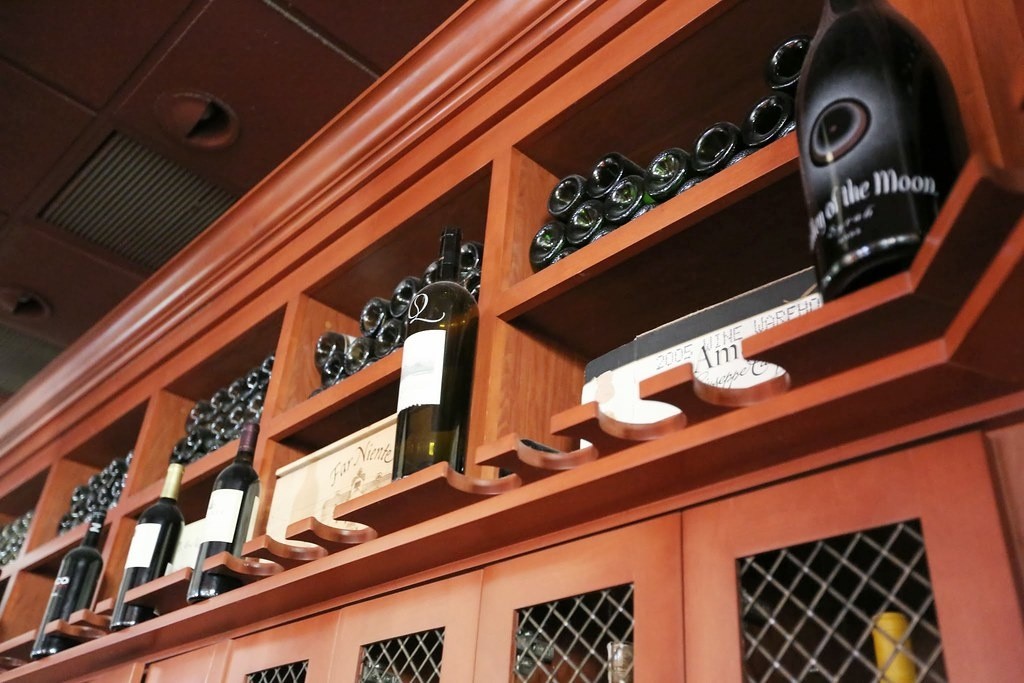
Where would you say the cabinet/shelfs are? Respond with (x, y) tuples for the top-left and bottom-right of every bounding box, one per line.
(0, 0), (1024, 683)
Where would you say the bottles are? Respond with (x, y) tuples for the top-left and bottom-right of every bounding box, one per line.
(608, 642), (633, 683)
(529, 0), (959, 303)
(307, 227), (484, 475)
(871, 613), (917, 683)
(0, 353), (275, 660)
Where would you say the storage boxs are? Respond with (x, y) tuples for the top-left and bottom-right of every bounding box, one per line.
(580, 264), (823, 449)
(262, 412), (398, 562)
